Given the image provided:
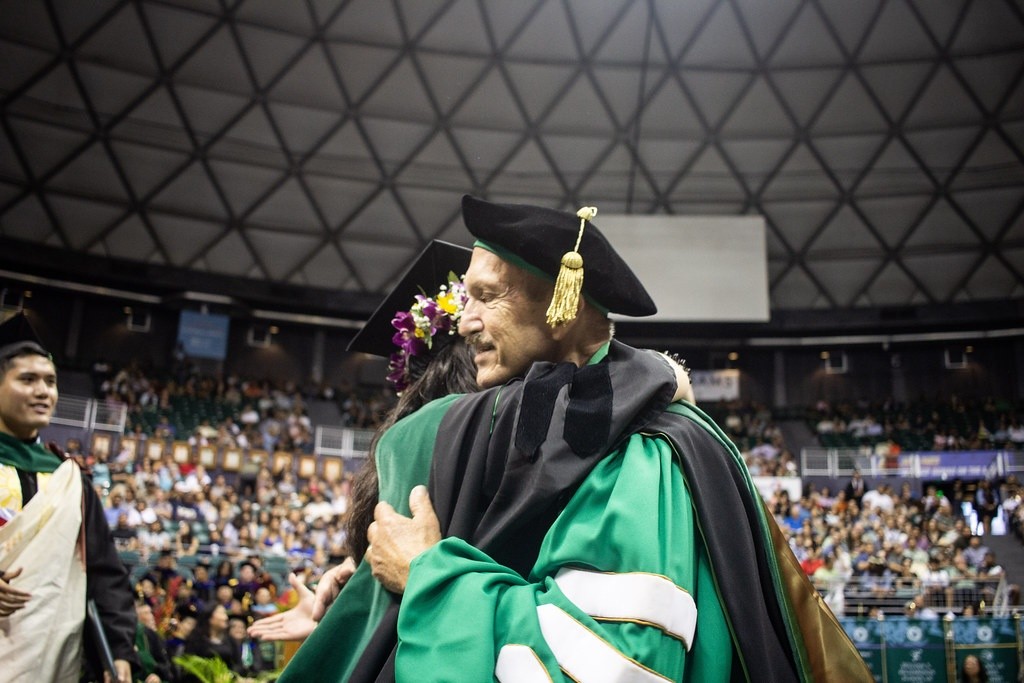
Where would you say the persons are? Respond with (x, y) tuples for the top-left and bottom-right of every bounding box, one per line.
(274, 233), (697, 683)
(0, 342), (137, 683)
(310, 196), (813, 683)
(1, 296), (1024, 682)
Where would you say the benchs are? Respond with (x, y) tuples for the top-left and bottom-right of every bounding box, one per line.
(0, 337), (1024, 664)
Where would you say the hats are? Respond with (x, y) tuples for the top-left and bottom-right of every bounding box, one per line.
(462, 190), (660, 328)
(347, 238), (486, 359)
